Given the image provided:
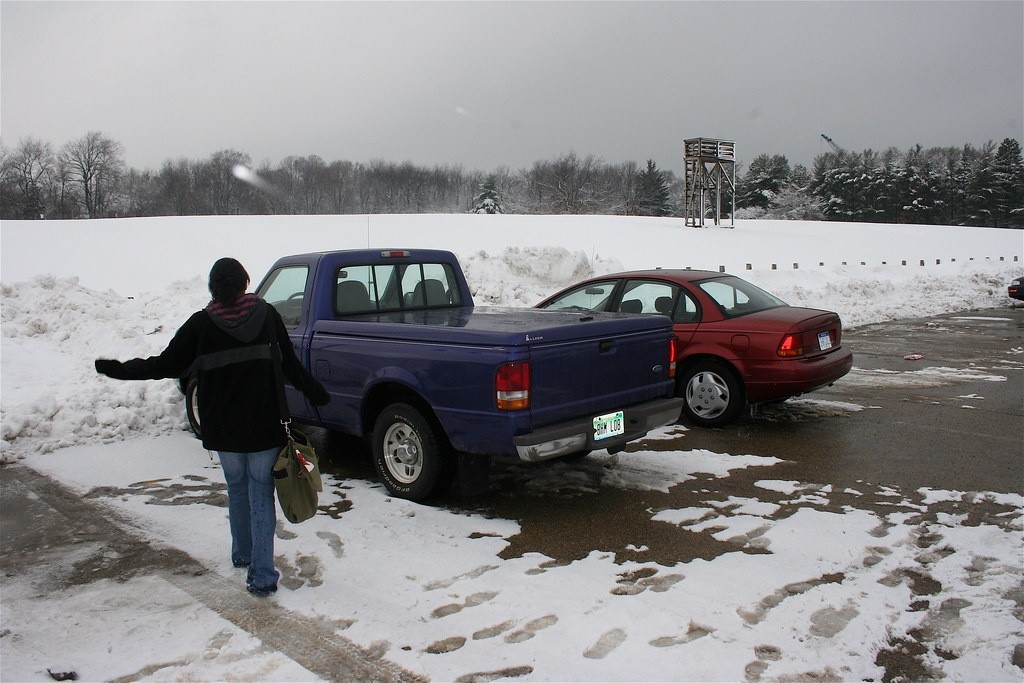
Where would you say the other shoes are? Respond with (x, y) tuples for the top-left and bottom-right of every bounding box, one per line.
(247, 583), (277, 598)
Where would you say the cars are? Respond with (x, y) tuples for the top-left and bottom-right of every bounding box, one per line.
(526, 269), (855, 429)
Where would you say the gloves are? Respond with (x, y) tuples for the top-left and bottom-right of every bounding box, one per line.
(311, 393), (331, 408)
(94, 359), (110, 375)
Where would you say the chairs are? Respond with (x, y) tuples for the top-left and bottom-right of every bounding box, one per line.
(337, 280), (373, 323)
(411, 278), (447, 325)
(620, 299), (643, 316)
(655, 296), (674, 313)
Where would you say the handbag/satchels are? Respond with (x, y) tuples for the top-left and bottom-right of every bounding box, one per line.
(271, 427), (323, 524)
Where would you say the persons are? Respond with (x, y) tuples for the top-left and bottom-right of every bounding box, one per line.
(95, 258), (332, 597)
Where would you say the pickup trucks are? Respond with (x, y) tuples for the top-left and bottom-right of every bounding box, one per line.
(174, 249), (685, 502)
